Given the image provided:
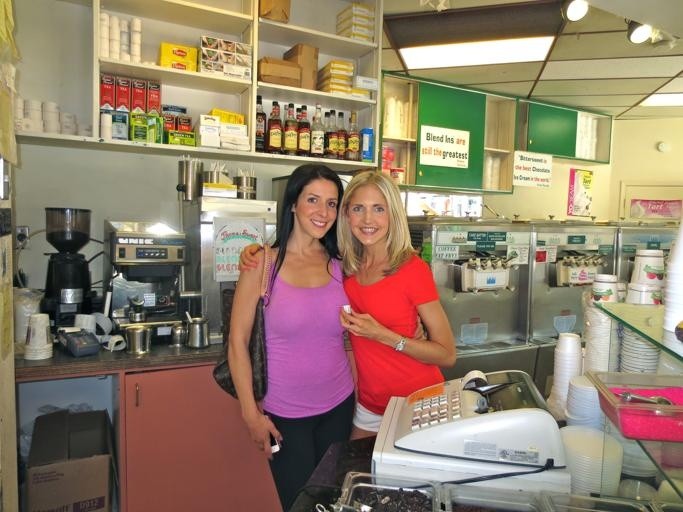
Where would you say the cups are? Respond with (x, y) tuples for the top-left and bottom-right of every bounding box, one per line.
(550, 248), (664, 426)
(177, 160), (257, 202)
(12, 98), (92, 137)
(126, 326), (151, 355)
(184, 318), (209, 349)
(661, 212), (683, 333)
(22, 312), (52, 360)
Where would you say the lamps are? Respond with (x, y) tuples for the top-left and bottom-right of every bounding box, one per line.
(622, 18), (655, 45)
(556, 1), (588, 25)
(383, 1), (574, 70)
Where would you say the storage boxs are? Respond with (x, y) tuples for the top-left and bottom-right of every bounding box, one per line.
(21, 401), (124, 510)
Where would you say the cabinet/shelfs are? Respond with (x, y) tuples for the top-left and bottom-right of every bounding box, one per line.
(584, 295), (681, 511)
(380, 70), (616, 197)
(110, 359), (283, 512)
(0, 0), (386, 174)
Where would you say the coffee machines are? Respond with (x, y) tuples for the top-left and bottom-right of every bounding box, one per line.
(100, 218), (189, 347)
(40, 208), (90, 341)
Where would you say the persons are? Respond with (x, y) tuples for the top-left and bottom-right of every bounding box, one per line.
(226, 163), (356, 511)
(239, 168), (456, 441)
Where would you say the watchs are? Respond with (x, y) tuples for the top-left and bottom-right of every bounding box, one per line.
(394, 337), (408, 352)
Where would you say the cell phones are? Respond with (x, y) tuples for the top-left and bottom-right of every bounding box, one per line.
(264, 415), (280, 453)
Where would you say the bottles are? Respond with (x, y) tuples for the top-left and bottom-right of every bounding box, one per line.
(170, 324), (186, 348)
(100, 12), (144, 65)
(126, 297), (146, 321)
(254, 95), (361, 161)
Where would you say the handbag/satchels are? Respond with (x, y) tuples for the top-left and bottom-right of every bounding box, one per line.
(212, 237), (275, 405)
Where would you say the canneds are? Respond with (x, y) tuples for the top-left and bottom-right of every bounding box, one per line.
(390, 168), (405, 183)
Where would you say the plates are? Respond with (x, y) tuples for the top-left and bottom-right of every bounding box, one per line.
(559, 379), (682, 506)
